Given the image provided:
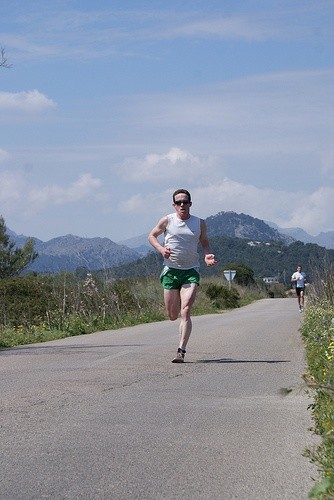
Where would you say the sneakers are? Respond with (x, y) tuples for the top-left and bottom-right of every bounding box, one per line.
(171, 348), (186, 363)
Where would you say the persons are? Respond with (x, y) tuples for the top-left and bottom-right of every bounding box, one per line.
(291, 266), (308, 313)
(148, 189), (217, 363)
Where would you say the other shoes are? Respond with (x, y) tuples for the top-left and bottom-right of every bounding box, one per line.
(299, 308), (304, 313)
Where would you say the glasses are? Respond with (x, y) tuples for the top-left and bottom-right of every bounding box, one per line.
(175, 200), (191, 205)
